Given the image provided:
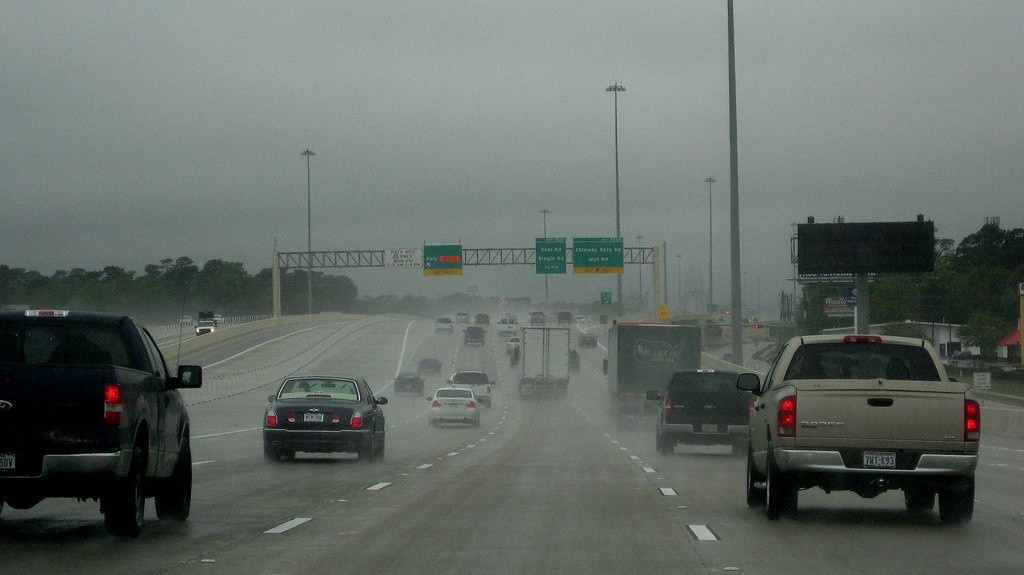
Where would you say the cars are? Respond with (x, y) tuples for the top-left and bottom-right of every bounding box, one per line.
(427, 387), (482, 428)
(432, 311), (598, 350)
(178, 314), (225, 337)
(392, 372), (426, 397)
(417, 357), (444, 377)
(262, 375), (388, 461)
(646, 368), (757, 455)
(446, 369), (496, 408)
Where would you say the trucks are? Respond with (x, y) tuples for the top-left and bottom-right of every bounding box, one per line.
(602, 319), (703, 431)
(517, 325), (571, 399)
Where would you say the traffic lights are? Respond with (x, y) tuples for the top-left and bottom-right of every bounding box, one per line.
(754, 324), (764, 329)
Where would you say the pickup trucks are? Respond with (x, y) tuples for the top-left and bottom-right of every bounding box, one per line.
(735, 333), (980, 526)
(0, 309), (203, 539)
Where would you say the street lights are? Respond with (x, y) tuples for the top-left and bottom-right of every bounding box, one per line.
(705, 175), (717, 314)
(676, 253), (684, 313)
(539, 208), (549, 301)
(300, 149), (315, 315)
(636, 235), (645, 305)
(606, 82), (627, 317)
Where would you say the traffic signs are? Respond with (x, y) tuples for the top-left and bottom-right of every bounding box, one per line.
(421, 245), (463, 278)
